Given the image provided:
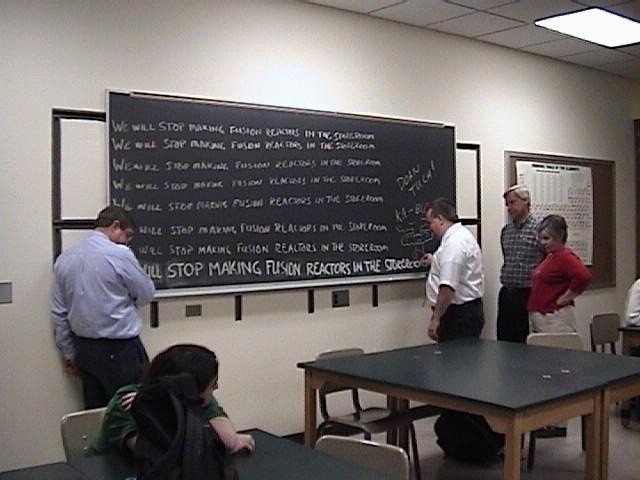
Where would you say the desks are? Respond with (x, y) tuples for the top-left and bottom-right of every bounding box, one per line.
(617, 327), (640, 354)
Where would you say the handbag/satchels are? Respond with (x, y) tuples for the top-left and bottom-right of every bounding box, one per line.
(432, 409), (505, 464)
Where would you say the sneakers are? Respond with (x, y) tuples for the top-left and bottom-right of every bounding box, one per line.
(534, 424), (566, 437)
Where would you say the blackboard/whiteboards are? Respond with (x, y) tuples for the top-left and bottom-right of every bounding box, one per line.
(106, 89), (459, 299)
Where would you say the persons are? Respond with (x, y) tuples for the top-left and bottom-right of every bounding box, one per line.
(48, 205), (155, 411)
(525, 214), (592, 438)
(617, 278), (640, 420)
(85, 344), (256, 457)
(419, 198), (484, 342)
(497, 184), (549, 342)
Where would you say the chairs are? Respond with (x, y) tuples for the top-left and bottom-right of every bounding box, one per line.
(590, 313), (620, 354)
(527, 333), (587, 470)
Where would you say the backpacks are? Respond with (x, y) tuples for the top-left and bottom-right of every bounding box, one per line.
(130, 377), (236, 475)
